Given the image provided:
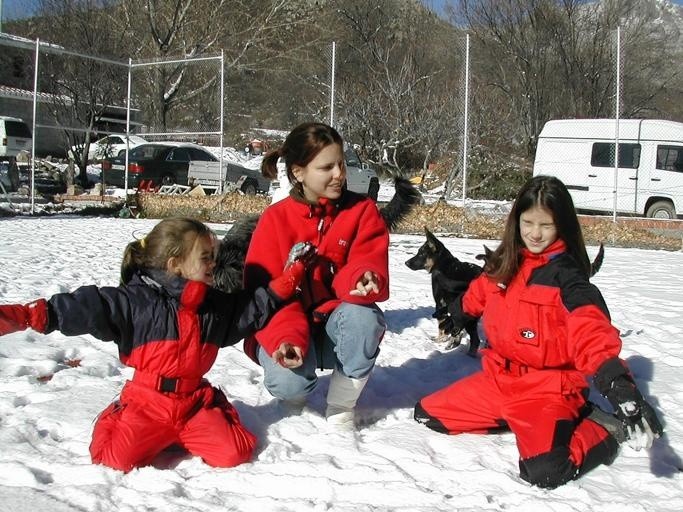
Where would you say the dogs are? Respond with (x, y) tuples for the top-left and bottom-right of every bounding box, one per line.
(474, 241), (606, 278)
(214, 174), (423, 294)
(404, 225), (487, 359)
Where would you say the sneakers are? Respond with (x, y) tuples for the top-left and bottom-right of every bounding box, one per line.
(584, 401), (630, 443)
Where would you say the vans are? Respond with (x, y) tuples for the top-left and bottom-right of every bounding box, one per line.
(0, 116), (31, 160)
(531, 117), (682, 222)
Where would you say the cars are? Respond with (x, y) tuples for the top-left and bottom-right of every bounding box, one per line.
(274, 144), (380, 202)
(98, 142), (218, 193)
(67, 134), (148, 163)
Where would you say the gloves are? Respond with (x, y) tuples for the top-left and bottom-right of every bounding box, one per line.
(592, 357), (663, 451)
(0, 298), (49, 336)
(432, 292), (480, 337)
(270, 241), (320, 301)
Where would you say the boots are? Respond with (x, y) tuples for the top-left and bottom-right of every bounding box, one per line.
(282, 394), (306, 415)
(325, 364), (369, 454)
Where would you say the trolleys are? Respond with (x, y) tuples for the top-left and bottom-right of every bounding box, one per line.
(187, 158), (271, 197)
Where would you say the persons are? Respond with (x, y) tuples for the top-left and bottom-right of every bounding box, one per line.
(244, 123), (389, 451)
(0, 217), (319, 474)
(415, 176), (663, 488)
